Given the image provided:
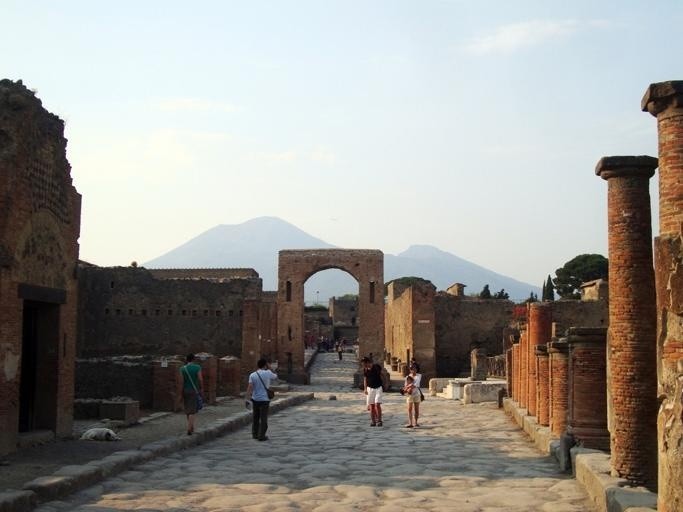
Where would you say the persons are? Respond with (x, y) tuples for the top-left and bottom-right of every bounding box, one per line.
(360, 357), (386, 427)
(245, 358), (278, 441)
(411, 358), (420, 373)
(399, 375), (412, 395)
(304, 334), (346, 361)
(403, 363), (422, 428)
(176, 353), (204, 436)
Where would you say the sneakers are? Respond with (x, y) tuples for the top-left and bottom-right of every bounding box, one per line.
(187, 427), (194, 435)
(251, 432), (271, 442)
(370, 418), (383, 426)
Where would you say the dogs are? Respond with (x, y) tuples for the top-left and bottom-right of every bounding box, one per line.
(80, 427), (122, 441)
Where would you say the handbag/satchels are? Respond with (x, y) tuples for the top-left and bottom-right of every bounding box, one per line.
(420, 395), (425, 402)
(196, 395), (204, 410)
(267, 390), (275, 398)
